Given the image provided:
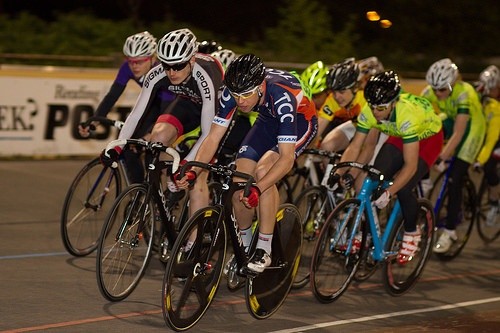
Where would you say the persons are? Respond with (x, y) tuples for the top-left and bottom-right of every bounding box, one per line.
(77, 32), (159, 199)
(207, 49), (238, 73)
(330, 68), (444, 264)
(195, 37), (222, 54)
(309, 56), (379, 245)
(300, 61), (329, 112)
(356, 55), (385, 89)
(418, 58), (486, 251)
(479, 64), (500, 101)
(170, 53), (319, 276)
(466, 80), (500, 226)
(100, 28), (225, 282)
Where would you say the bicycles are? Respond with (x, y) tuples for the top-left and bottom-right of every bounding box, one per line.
(161, 160), (304, 331)
(60, 122), (177, 257)
(310, 161), (436, 305)
(272, 146), (500, 291)
(95, 138), (227, 303)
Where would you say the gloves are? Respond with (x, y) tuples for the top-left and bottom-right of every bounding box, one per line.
(324, 173), (344, 193)
(373, 189), (392, 209)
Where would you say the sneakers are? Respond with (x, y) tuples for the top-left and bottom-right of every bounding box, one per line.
(245, 248), (271, 274)
(338, 233), (369, 252)
(486, 207), (498, 227)
(433, 231), (457, 253)
(224, 254), (239, 274)
(396, 226), (420, 264)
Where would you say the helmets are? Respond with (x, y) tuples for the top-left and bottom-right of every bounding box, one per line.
(479, 65), (499, 90)
(300, 61), (329, 94)
(123, 31), (157, 57)
(211, 49), (236, 72)
(157, 28), (198, 63)
(364, 70), (400, 104)
(327, 57), (360, 89)
(426, 58), (458, 89)
(197, 41), (221, 54)
(224, 53), (266, 93)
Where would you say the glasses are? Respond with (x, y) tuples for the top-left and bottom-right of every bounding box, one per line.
(129, 57), (150, 64)
(162, 54), (194, 71)
(228, 85), (263, 99)
(432, 88), (446, 91)
(368, 99), (394, 110)
